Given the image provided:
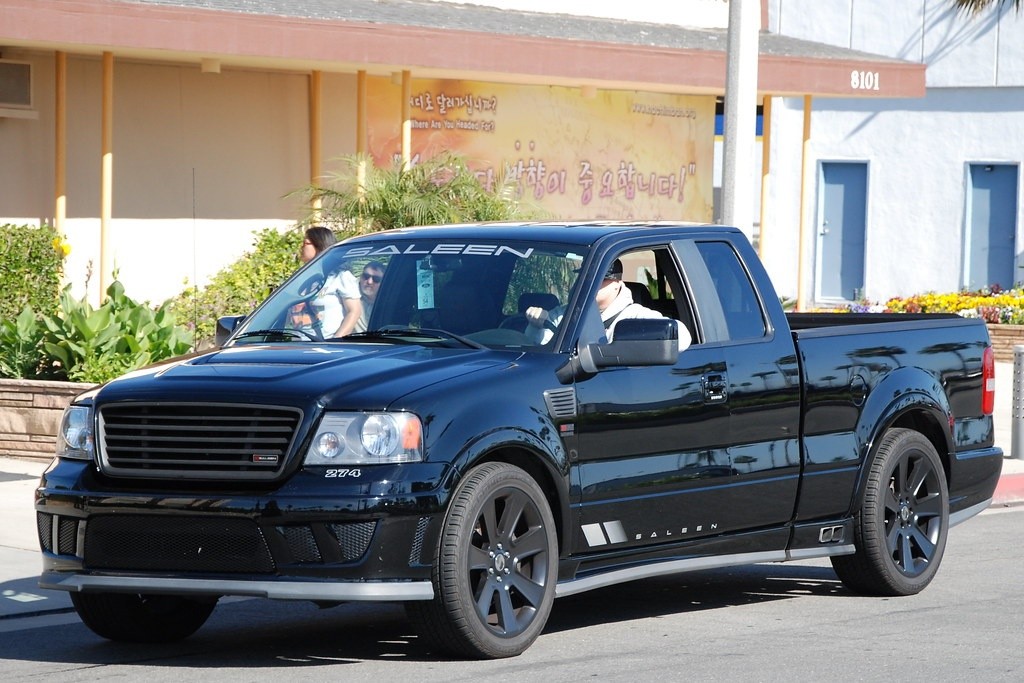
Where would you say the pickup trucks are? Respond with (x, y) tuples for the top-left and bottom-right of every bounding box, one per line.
(29, 222), (1005, 661)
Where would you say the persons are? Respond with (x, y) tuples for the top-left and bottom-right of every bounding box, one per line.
(524, 256), (692, 352)
(288, 227), (387, 341)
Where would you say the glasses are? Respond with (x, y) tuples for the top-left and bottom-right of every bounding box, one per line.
(303, 240), (314, 247)
(361, 273), (382, 283)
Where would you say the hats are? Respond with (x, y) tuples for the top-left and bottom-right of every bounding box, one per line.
(572, 258), (623, 281)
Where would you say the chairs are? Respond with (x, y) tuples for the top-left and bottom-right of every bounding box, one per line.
(499, 292), (559, 332)
(623, 281), (653, 310)
